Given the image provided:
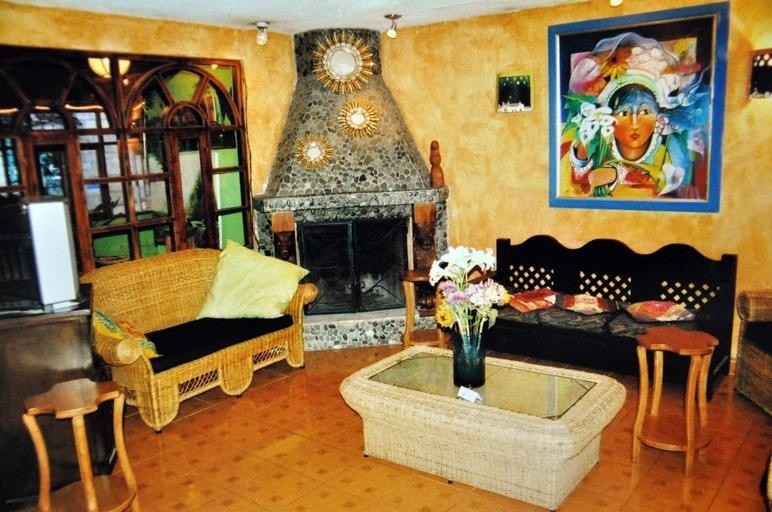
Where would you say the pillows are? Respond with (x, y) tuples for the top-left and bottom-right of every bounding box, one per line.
(507, 288), (561, 314)
(196, 238), (311, 319)
(91, 308), (165, 367)
(619, 298), (695, 325)
(543, 292), (617, 315)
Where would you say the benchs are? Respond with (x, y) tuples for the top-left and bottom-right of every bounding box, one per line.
(75, 248), (319, 432)
(485, 235), (736, 401)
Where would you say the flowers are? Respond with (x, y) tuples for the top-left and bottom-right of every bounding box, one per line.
(569, 100), (617, 198)
(428, 244), (515, 363)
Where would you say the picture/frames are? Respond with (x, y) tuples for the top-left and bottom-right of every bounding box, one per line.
(492, 68), (537, 116)
(744, 44), (772, 99)
(548, 1), (728, 210)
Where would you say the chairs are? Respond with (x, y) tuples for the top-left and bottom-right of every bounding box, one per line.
(734, 287), (772, 415)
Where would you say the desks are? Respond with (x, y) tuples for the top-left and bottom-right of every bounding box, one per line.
(21, 376), (150, 510)
(399, 266), (451, 354)
(633, 324), (717, 476)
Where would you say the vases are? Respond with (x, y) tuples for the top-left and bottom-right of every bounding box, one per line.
(441, 307), (489, 389)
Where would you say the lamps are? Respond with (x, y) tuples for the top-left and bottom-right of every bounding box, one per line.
(382, 12), (406, 39)
(86, 56), (133, 81)
(255, 19), (271, 48)
